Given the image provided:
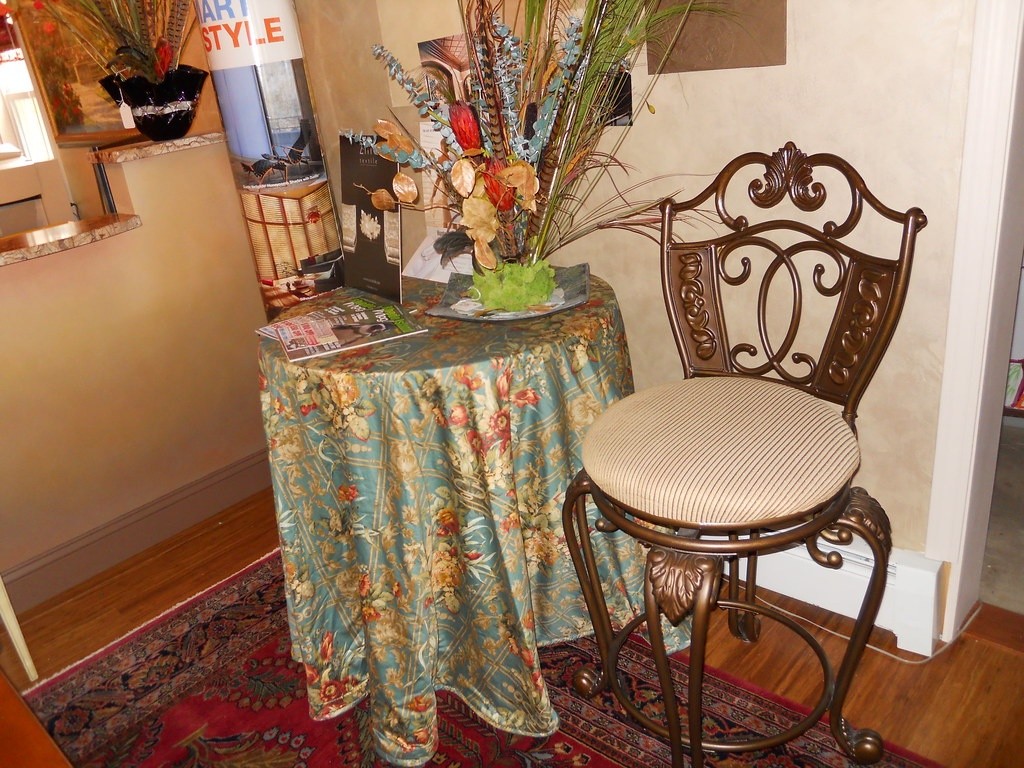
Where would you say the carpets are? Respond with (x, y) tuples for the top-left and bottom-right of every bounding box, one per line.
(18, 544), (950, 768)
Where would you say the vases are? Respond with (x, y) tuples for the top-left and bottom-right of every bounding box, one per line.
(98, 64), (209, 141)
(472, 258), (553, 312)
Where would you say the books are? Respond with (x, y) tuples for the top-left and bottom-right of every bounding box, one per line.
(255, 296), (420, 345)
(275, 303), (428, 362)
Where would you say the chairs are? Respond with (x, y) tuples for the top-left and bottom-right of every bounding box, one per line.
(241, 117), (314, 185)
(559, 139), (926, 764)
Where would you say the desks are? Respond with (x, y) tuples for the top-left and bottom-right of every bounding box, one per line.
(260, 266), (655, 767)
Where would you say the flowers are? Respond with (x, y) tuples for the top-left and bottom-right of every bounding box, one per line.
(33, 0), (198, 83)
(352, 0), (749, 314)
